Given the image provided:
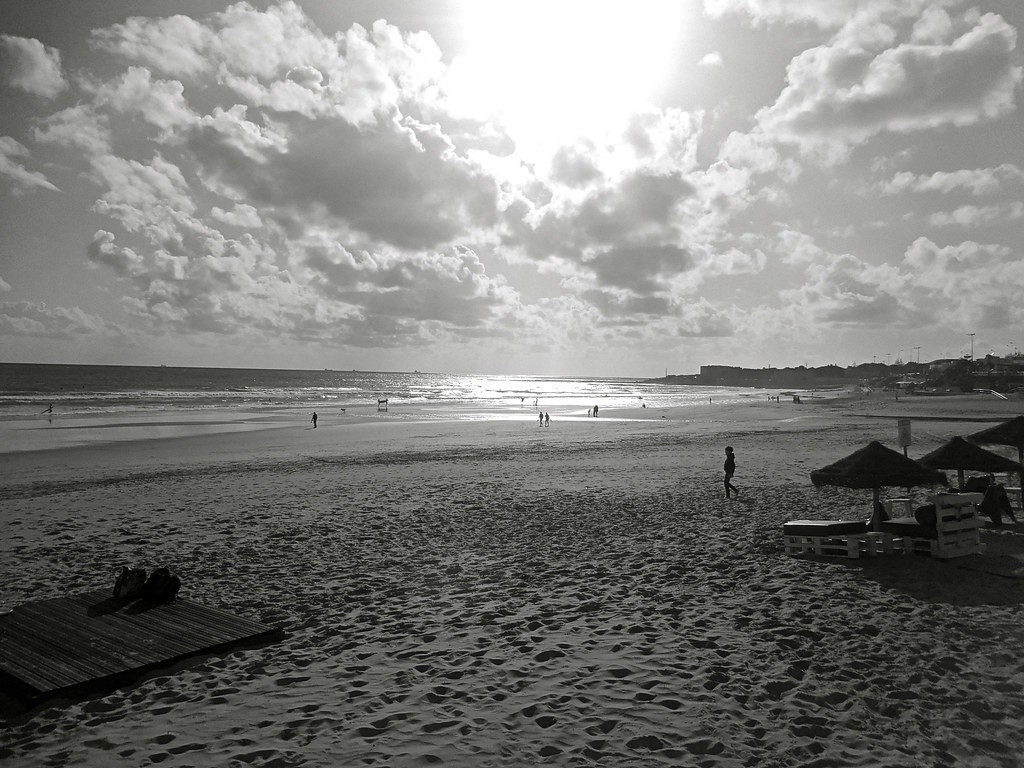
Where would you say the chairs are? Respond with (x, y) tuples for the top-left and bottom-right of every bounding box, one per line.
(915, 473), (1016, 527)
(880, 491), (987, 559)
(784, 520), (885, 559)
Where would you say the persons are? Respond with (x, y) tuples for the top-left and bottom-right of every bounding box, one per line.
(588, 409), (591, 417)
(724, 446), (740, 498)
(867, 500), (888, 533)
(48, 403), (53, 413)
(312, 412), (318, 428)
(539, 411), (543, 427)
(593, 405), (598, 417)
(544, 412), (550, 427)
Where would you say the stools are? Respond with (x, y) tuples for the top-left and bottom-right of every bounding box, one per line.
(885, 498), (913, 520)
(1004, 487), (1022, 509)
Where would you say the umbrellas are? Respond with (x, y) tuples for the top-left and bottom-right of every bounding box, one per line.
(811, 441), (942, 524)
(916, 434), (1024, 494)
(965, 415), (1024, 490)
